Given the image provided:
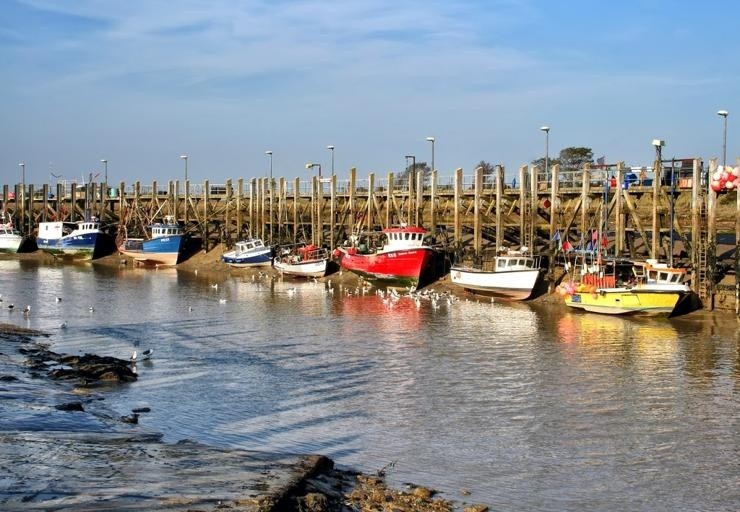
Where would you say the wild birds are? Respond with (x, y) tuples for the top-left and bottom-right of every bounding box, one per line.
(142, 347), (154, 358)
(21, 305), (32, 316)
(130, 350), (138, 361)
(59, 320), (68, 329)
(87, 304), (94, 311)
(7, 303), (14, 310)
(55, 297), (62, 302)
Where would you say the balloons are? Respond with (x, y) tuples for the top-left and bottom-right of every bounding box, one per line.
(710, 165), (739, 193)
(555, 281), (577, 297)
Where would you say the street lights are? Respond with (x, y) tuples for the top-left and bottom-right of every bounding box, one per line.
(306, 163), (321, 176)
(404, 155), (416, 191)
(425, 136), (434, 169)
(649, 138), (664, 163)
(539, 126), (549, 186)
(100, 160), (108, 184)
(18, 163), (26, 184)
(715, 110), (728, 169)
(180, 155), (187, 182)
(266, 151), (273, 177)
(325, 145), (333, 174)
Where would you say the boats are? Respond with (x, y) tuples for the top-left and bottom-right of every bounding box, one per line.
(0, 216), (198, 269)
(331, 221), (441, 295)
(220, 235), (270, 269)
(444, 251), (542, 302)
(269, 242), (330, 279)
(552, 181), (695, 320)
